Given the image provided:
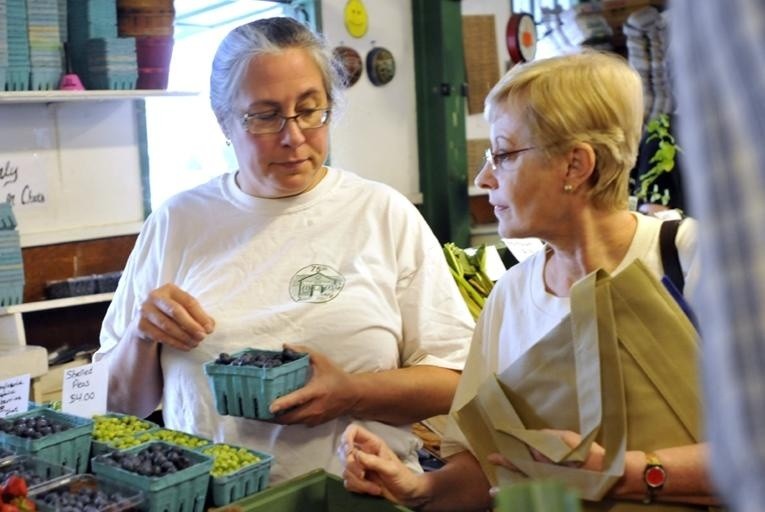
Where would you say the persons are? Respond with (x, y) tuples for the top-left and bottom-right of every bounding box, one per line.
(335, 47), (716, 512)
(90, 15), (477, 484)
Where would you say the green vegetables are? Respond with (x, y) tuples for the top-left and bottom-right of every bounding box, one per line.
(444, 240), (494, 323)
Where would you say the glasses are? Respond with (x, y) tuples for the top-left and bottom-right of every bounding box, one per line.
(485, 141), (569, 171)
(223, 100), (334, 136)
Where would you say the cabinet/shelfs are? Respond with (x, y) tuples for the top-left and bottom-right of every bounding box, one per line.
(0, 85), (200, 415)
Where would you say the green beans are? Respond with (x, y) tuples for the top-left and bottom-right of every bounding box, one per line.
(91, 415), (260, 483)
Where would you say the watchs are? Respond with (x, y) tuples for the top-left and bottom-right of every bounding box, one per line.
(641, 451), (667, 506)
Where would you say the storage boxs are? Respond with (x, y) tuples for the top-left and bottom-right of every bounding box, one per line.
(209, 469), (414, 512)
